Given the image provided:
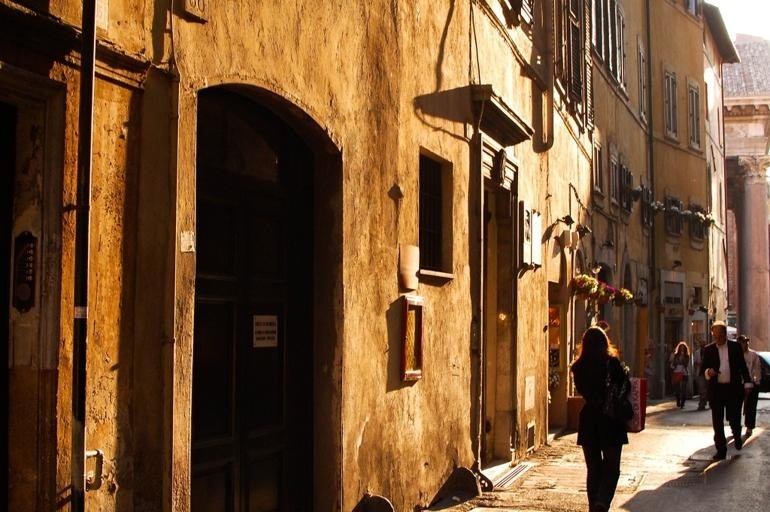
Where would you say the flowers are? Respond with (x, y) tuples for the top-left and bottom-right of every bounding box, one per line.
(573, 275), (633, 333)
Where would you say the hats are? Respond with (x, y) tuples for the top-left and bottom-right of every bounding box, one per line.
(737, 335), (749, 344)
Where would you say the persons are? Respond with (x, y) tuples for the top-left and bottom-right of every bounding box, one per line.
(701, 319), (755, 458)
(570, 325), (636, 511)
(732, 334), (763, 437)
(671, 341), (690, 408)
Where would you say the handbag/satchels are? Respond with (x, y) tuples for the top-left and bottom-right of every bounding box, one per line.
(672, 372), (682, 384)
(604, 394), (634, 422)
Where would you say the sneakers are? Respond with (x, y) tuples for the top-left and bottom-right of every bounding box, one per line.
(713, 451), (726, 460)
(698, 405), (705, 411)
(676, 401), (685, 408)
(747, 427), (752, 435)
(734, 436), (743, 451)
